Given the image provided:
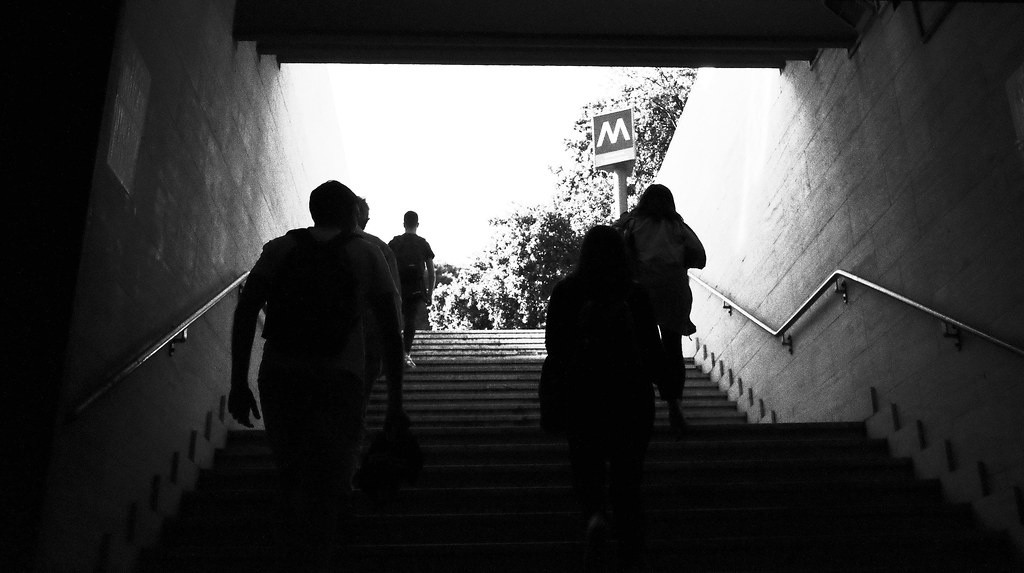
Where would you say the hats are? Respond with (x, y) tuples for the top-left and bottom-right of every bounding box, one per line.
(640, 186), (675, 216)
(309, 181), (358, 220)
(404, 212), (417, 227)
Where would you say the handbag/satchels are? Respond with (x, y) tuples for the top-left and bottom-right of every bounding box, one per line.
(629, 257), (680, 307)
(402, 263), (420, 301)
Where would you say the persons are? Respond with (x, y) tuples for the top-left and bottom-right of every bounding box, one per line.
(538, 184), (708, 535)
(229, 181), (435, 533)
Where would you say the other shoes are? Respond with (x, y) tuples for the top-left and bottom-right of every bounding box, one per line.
(403, 353), (416, 368)
(582, 515), (608, 573)
(669, 406), (684, 426)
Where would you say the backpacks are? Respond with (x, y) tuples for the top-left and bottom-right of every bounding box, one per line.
(258, 228), (362, 365)
